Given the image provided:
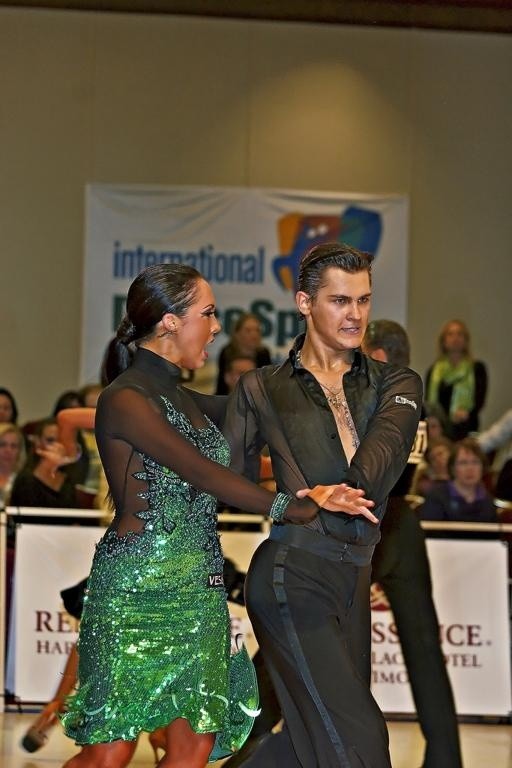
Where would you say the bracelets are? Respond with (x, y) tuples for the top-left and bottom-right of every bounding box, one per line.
(270, 493), (292, 524)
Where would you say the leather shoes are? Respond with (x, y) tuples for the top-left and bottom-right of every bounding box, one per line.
(22, 729), (46, 754)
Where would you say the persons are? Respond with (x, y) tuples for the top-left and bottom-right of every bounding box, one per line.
(422, 322), (490, 437)
(479, 405), (512, 501)
(412, 409), (497, 521)
(22, 405), (171, 764)
(219, 241), (424, 768)
(0, 385), (101, 547)
(259, 317), (462, 768)
(215, 312), (273, 398)
(220, 355), (257, 397)
(61, 263), (353, 768)
